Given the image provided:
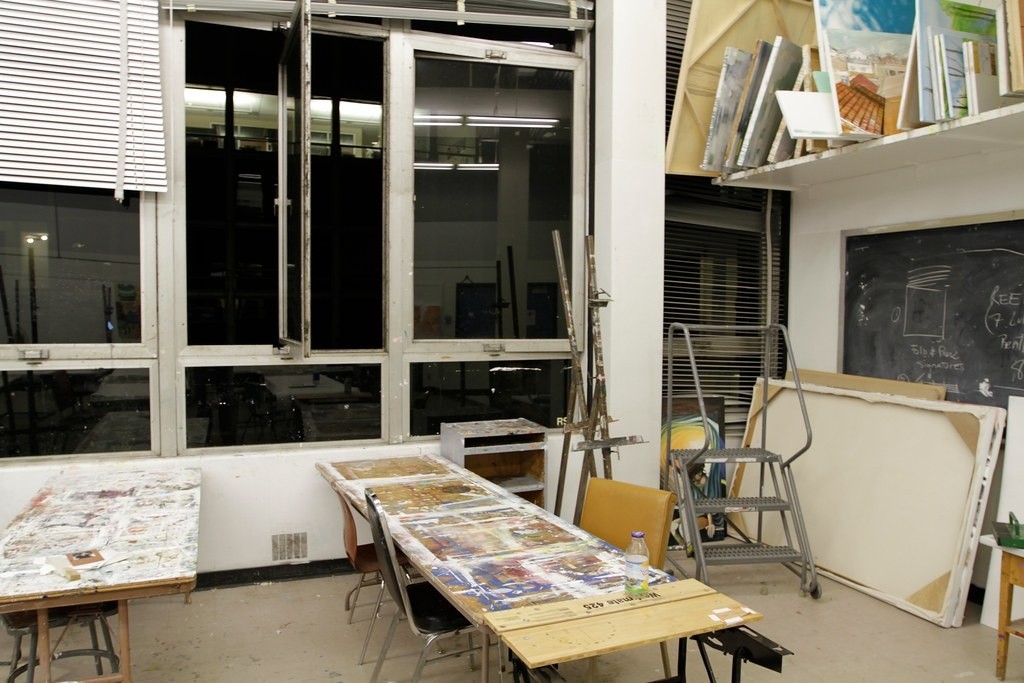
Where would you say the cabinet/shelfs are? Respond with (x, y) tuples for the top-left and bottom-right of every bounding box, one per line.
(441, 417), (548, 512)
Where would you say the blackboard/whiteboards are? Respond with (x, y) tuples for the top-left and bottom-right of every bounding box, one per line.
(837, 208), (1024, 411)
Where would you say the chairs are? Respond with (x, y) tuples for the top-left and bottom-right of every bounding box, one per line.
(230, 370), (288, 445)
(329, 481), (444, 666)
(581, 477), (677, 683)
(49, 370), (106, 454)
(363, 488), (491, 683)
(0, 600), (119, 683)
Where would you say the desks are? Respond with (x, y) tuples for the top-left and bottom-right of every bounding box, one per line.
(90, 368), (150, 411)
(71, 412), (208, 454)
(315, 453), (677, 683)
(0, 467), (200, 683)
(980, 535), (1024, 682)
(302, 401), (382, 441)
(263, 374), (361, 413)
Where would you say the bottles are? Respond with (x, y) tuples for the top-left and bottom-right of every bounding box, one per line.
(623, 531), (651, 601)
